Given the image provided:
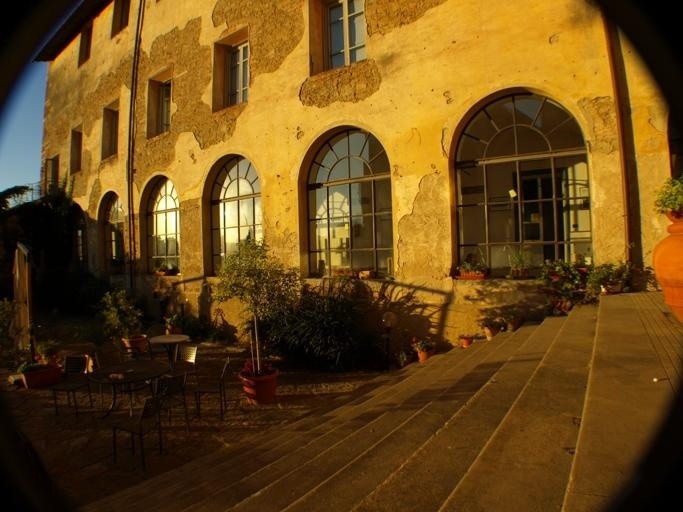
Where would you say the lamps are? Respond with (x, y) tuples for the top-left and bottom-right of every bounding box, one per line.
(379, 310), (399, 370)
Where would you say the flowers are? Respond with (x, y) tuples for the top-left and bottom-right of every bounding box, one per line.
(410, 334), (437, 352)
(18, 345), (60, 371)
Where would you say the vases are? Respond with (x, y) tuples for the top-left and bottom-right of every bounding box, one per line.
(460, 335), (473, 348)
(22, 367), (64, 390)
(417, 349), (434, 362)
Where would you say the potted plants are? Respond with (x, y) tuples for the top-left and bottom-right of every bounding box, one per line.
(162, 312), (179, 335)
(475, 302), (528, 339)
(574, 254), (591, 273)
(92, 290), (149, 355)
(601, 260), (662, 296)
(453, 248), (490, 281)
(204, 232), (305, 404)
(651, 174), (683, 325)
(503, 240), (540, 278)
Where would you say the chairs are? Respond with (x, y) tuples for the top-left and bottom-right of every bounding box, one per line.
(48, 334), (235, 473)
